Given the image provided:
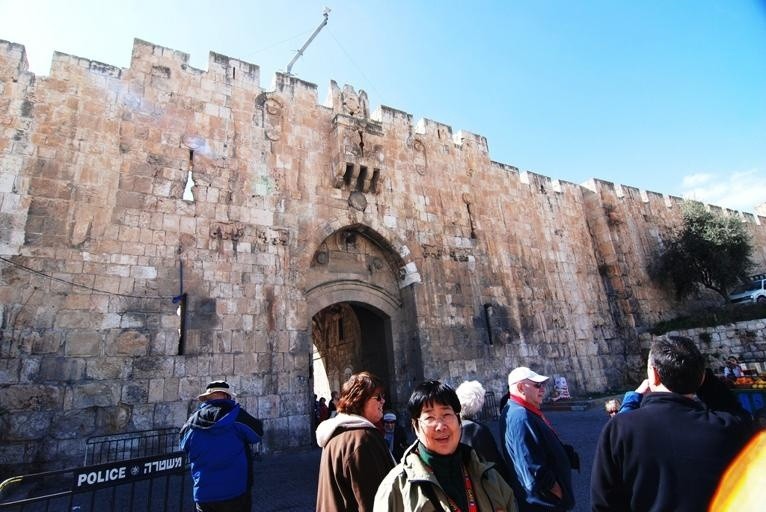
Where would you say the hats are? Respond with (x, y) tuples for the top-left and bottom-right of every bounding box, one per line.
(384, 413), (397, 422)
(199, 380), (232, 402)
(508, 367), (550, 384)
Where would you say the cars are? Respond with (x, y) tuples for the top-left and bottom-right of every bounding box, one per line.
(723, 279), (766, 306)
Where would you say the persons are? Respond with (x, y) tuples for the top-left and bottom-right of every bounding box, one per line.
(454, 380), (527, 512)
(382, 409), (409, 464)
(589, 335), (755, 512)
(605, 399), (623, 418)
(314, 372), (397, 512)
(724, 356), (745, 381)
(315, 389), (340, 423)
(371, 382), (520, 511)
(617, 379), (650, 415)
(498, 366), (577, 511)
(178, 380), (264, 512)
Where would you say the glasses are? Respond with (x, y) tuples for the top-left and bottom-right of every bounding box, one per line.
(370, 392), (385, 401)
(414, 412), (461, 426)
(609, 410), (618, 414)
